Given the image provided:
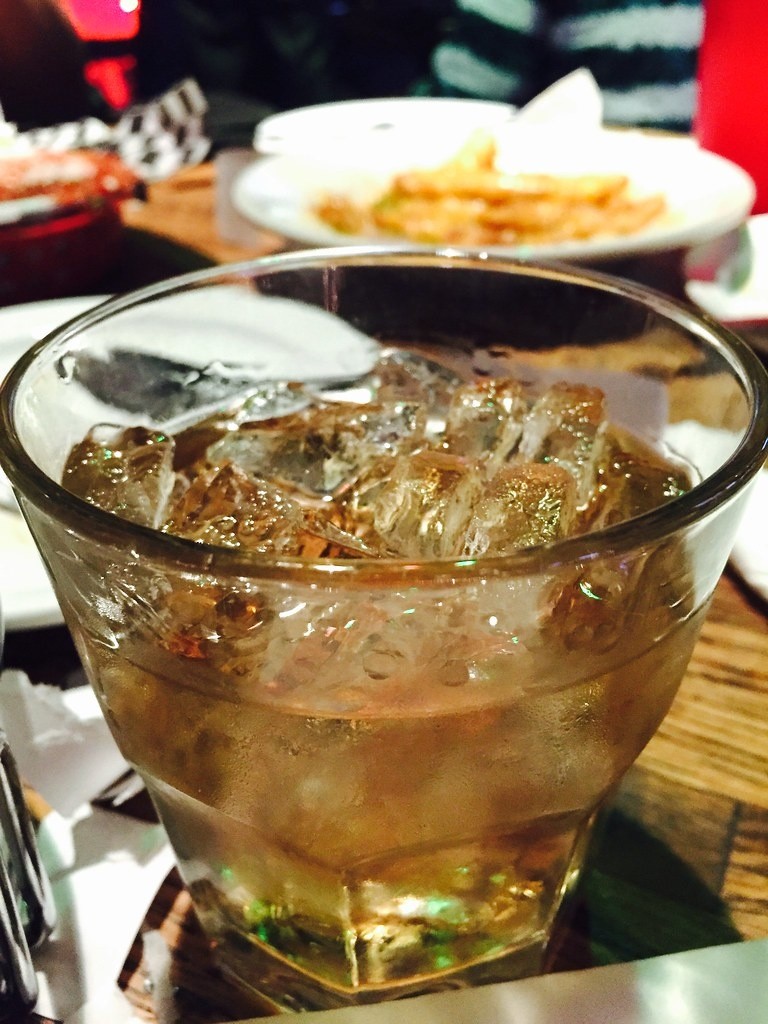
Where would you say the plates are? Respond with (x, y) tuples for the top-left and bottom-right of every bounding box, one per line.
(0, 291), (389, 627)
(233, 122), (755, 259)
(256, 98), (511, 153)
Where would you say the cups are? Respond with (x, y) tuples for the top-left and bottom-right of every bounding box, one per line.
(0, 244), (766, 1008)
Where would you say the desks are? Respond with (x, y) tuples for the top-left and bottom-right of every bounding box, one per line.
(23, 169), (767, 1024)
